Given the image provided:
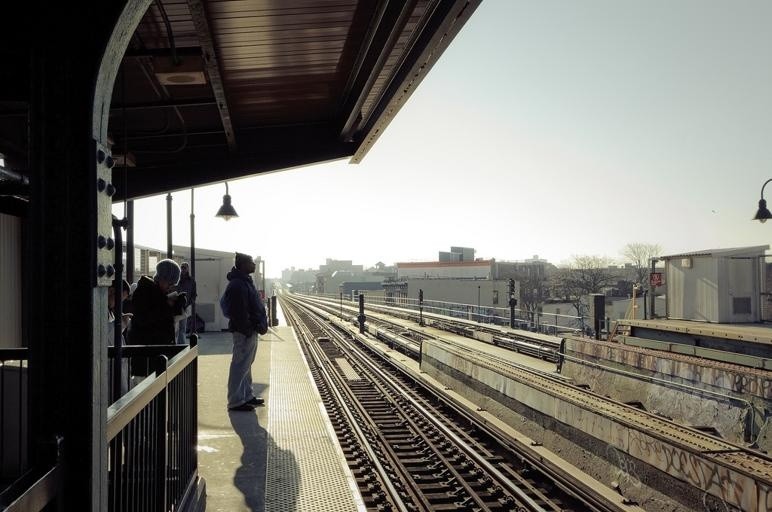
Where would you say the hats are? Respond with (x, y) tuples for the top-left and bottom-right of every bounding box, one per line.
(156, 259), (181, 283)
(179, 261), (189, 270)
(235, 252), (251, 267)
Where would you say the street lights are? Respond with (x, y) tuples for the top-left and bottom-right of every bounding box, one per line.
(190, 179), (240, 331)
(752, 179), (772, 224)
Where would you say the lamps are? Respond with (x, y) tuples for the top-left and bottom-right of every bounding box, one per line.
(214, 179), (238, 222)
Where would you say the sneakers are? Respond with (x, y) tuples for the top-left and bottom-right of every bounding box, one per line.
(246, 396), (265, 405)
(228, 402), (255, 412)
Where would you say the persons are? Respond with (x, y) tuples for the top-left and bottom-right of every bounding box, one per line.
(227, 252), (268, 411)
(107, 258), (197, 396)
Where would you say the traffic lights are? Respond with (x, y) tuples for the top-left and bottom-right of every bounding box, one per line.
(510, 279), (516, 295)
